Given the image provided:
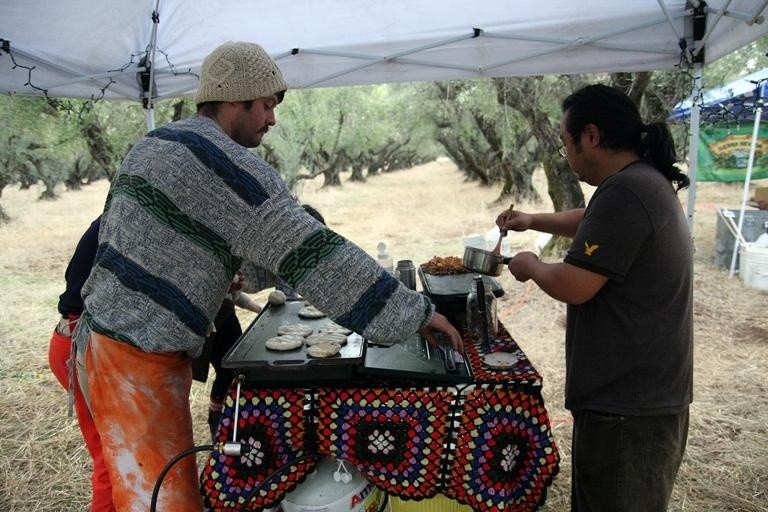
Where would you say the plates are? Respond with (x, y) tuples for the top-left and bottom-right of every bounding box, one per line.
(481, 351), (517, 369)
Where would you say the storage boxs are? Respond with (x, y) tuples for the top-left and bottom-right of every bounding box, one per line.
(418, 263), (505, 326)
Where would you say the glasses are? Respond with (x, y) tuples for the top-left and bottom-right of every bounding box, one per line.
(556, 135), (583, 159)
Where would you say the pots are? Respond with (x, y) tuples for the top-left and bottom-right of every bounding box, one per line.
(462, 246), (529, 276)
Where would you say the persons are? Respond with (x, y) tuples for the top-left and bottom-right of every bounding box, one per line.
(63, 38), (467, 511)
(198, 201), (328, 442)
(492, 79), (698, 512)
(46, 204), (118, 511)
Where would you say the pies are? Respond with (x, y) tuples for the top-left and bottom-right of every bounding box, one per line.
(422, 256), (471, 275)
(266, 305), (352, 358)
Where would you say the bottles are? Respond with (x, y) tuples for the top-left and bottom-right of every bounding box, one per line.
(465, 278), (499, 343)
(377, 242), (417, 293)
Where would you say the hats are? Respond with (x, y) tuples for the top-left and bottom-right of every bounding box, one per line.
(194, 40), (288, 104)
